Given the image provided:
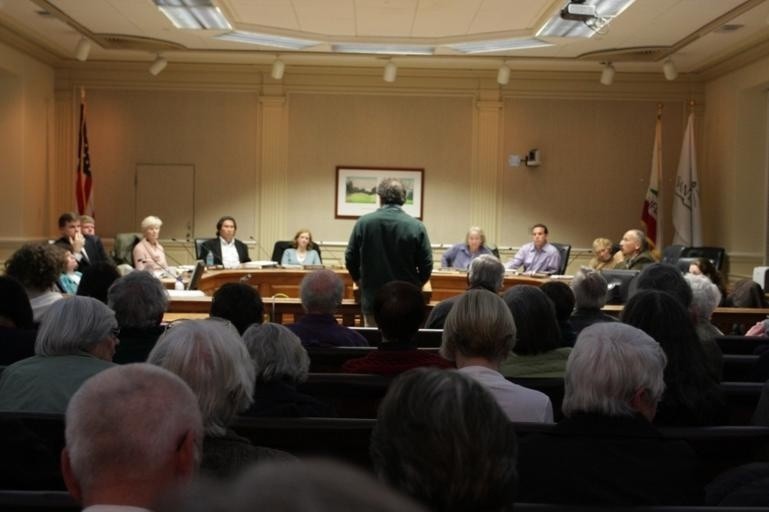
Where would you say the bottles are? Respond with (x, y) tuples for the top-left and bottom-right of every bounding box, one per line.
(205, 250), (214, 267)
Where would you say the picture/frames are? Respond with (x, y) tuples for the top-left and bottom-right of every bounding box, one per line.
(333, 162), (426, 226)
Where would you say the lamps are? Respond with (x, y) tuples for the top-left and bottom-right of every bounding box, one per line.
(379, 55), (399, 85)
(147, 51), (168, 77)
(267, 51), (287, 81)
(492, 58), (514, 87)
(75, 30), (95, 64)
(659, 52), (679, 82)
(596, 61), (617, 88)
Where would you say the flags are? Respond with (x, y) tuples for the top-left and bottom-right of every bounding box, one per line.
(75, 101), (96, 219)
(639, 116), (662, 260)
(671, 111), (701, 249)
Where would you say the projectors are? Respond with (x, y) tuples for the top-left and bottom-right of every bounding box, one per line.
(560, 4), (596, 21)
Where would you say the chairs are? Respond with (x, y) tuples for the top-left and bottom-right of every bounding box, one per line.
(0, 233), (768, 512)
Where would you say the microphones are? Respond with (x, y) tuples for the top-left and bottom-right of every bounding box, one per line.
(497, 247), (513, 250)
(319, 240), (343, 269)
(565, 250), (593, 269)
(134, 233), (273, 290)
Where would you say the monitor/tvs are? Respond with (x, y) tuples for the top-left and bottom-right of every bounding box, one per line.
(601, 268), (639, 302)
